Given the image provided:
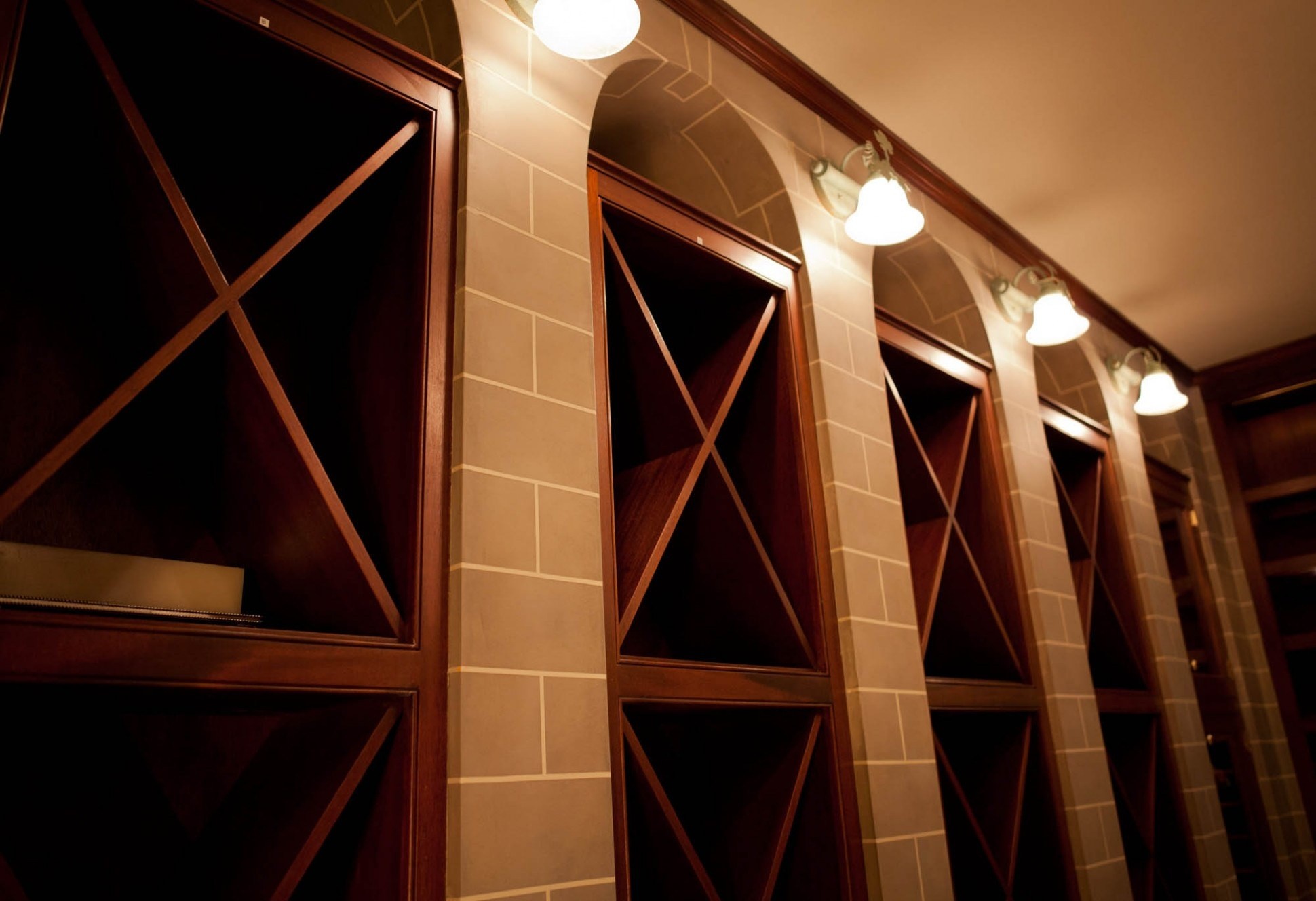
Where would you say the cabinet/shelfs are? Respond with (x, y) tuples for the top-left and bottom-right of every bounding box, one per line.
(1, 0), (1316, 901)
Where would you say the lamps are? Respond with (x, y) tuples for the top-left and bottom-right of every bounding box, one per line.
(508, 0), (640, 60)
(811, 141), (924, 246)
(1106, 346), (1189, 415)
(991, 265), (1089, 345)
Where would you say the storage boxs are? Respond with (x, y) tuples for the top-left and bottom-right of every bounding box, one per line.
(0, 542), (245, 612)
